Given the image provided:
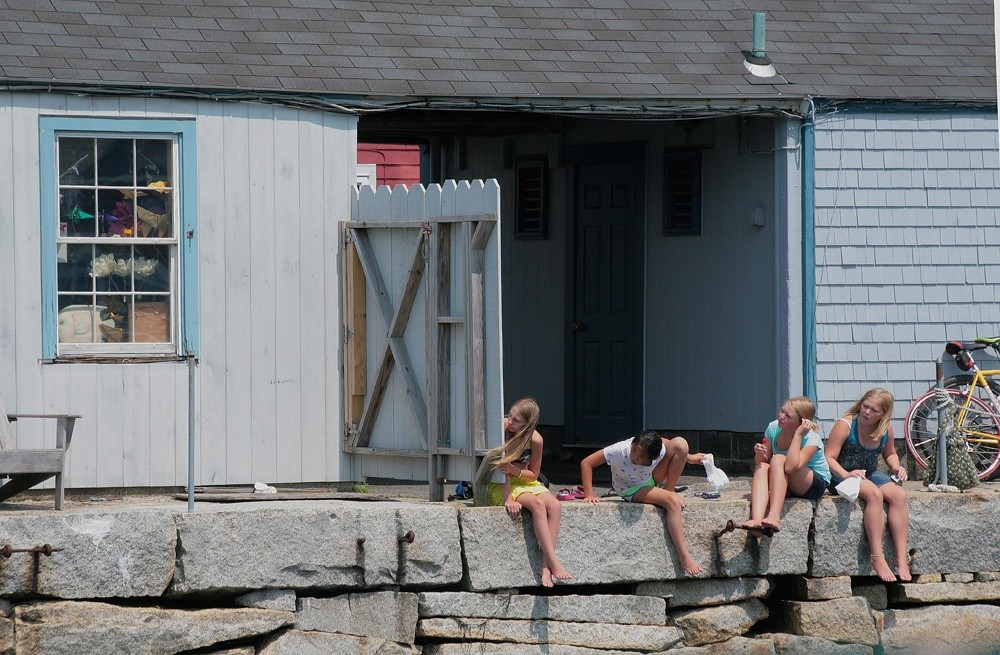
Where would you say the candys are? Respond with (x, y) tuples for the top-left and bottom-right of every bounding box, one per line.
(762, 438), (766, 444)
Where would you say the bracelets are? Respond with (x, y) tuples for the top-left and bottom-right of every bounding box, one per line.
(516, 469), (523, 479)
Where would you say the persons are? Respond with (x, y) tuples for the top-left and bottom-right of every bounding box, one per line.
(741, 398), (832, 537)
(824, 388), (913, 583)
(482, 397), (571, 587)
(580, 430), (707, 575)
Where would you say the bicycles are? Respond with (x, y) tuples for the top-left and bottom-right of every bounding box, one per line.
(900, 336), (999, 483)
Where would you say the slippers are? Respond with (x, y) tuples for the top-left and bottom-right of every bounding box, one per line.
(557, 488), (575, 500)
(601, 487), (617, 497)
(571, 485), (597, 499)
